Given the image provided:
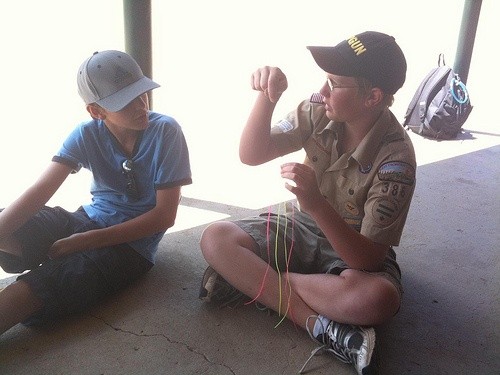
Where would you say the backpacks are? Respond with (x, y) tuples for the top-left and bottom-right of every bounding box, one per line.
(403, 53), (474, 142)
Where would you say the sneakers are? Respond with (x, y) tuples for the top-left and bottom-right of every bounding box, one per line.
(198, 265), (242, 303)
(298, 315), (377, 375)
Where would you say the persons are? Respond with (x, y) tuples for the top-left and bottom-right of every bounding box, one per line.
(199, 29), (417, 375)
(0, 49), (193, 336)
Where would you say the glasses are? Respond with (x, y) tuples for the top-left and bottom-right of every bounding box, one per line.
(121, 159), (139, 203)
(325, 77), (362, 92)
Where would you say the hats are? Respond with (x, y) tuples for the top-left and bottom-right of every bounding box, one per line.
(76, 50), (160, 113)
(306, 31), (407, 96)
